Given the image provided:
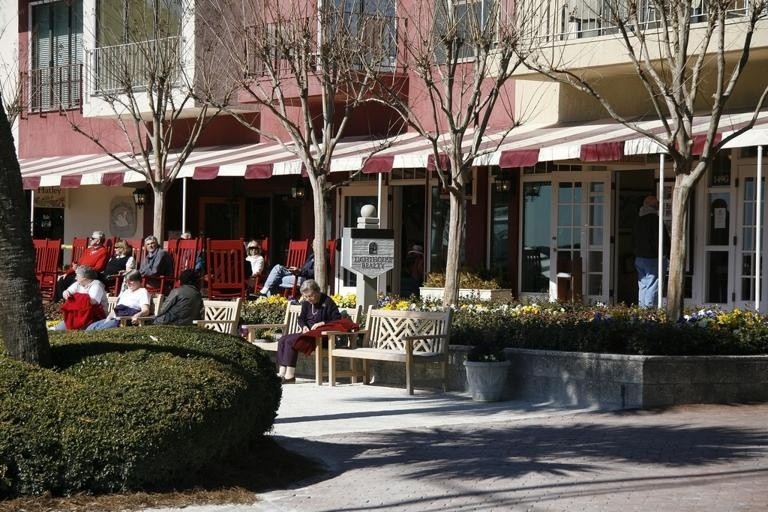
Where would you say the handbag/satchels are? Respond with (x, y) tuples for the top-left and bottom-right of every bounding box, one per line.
(114, 304), (141, 327)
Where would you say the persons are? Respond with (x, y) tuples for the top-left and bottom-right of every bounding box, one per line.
(633, 194), (669, 310)
(55, 230), (108, 304)
(243, 239), (264, 277)
(85, 240), (135, 292)
(46, 264), (109, 331)
(148, 267), (204, 325)
(248, 239), (314, 298)
(407, 244), (425, 282)
(85, 268), (151, 330)
(272, 278), (341, 384)
(137, 236), (174, 296)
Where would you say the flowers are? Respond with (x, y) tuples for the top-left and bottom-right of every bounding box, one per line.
(376, 295), (417, 311)
(255, 293), (356, 307)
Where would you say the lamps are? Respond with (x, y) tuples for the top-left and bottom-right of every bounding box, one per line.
(524, 168), (545, 202)
(290, 174), (311, 201)
(493, 169), (513, 204)
(132, 186), (151, 210)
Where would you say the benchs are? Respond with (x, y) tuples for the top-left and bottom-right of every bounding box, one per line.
(322, 304), (452, 395)
(106, 292), (168, 330)
(244, 301), (363, 385)
(137, 293), (241, 340)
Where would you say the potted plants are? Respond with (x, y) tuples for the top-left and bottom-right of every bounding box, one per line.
(417, 271), (514, 307)
(462, 340), (514, 401)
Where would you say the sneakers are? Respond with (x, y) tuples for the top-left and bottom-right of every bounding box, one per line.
(249, 291), (267, 298)
(281, 376), (295, 384)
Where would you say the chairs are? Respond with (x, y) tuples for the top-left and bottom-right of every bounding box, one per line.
(238, 239), (273, 294)
(206, 238), (247, 301)
(60, 237), (204, 301)
(31, 238), (62, 303)
(277, 239), (310, 293)
(292, 239), (337, 294)
